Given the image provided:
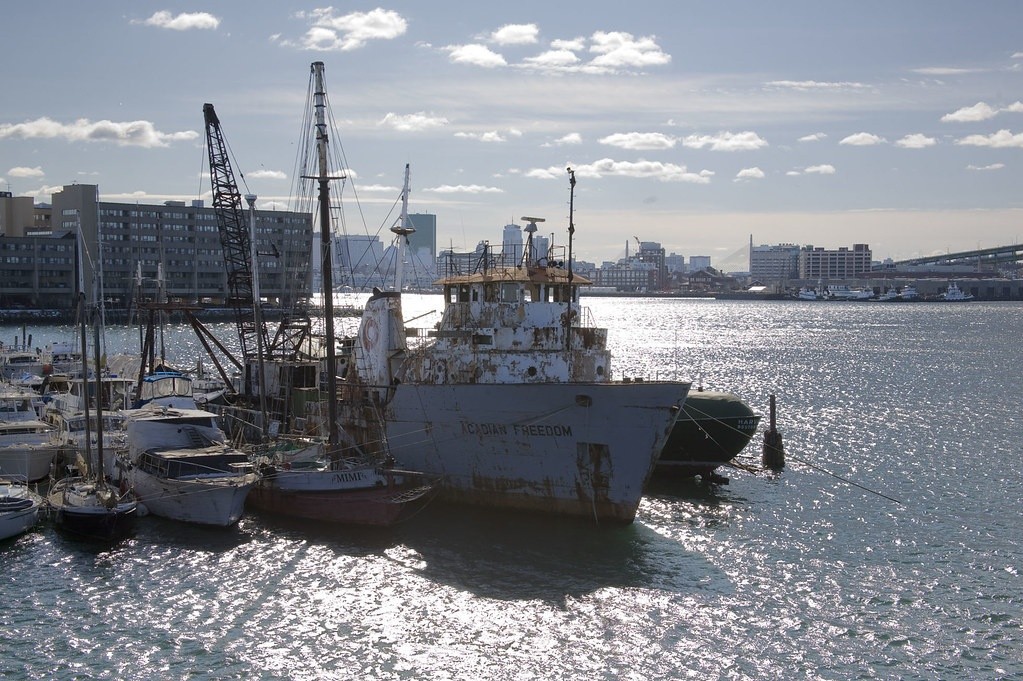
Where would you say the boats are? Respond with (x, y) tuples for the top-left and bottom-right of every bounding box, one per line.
(788, 273), (977, 303)
(652, 387), (763, 486)
(225, 163), (695, 528)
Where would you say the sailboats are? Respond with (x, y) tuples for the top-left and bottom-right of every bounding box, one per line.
(0, 60), (430, 549)
